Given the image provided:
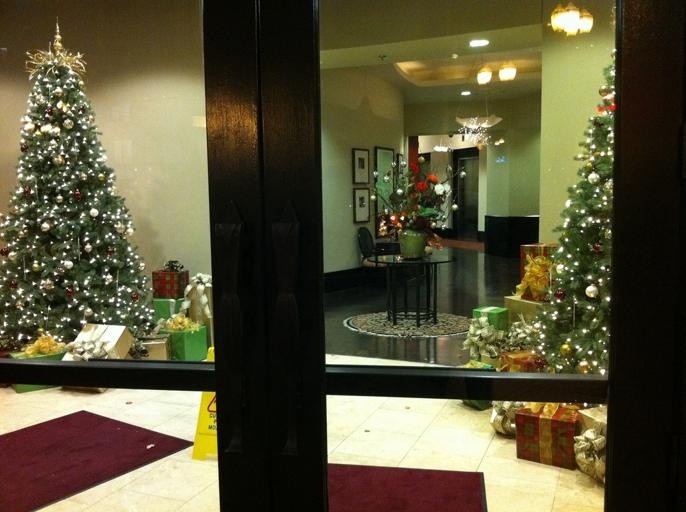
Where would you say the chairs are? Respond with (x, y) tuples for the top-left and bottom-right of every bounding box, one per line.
(356, 226), (396, 291)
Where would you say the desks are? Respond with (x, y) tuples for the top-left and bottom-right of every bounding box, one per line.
(366, 253), (454, 327)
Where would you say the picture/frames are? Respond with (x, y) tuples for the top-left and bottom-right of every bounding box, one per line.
(351, 145), (404, 224)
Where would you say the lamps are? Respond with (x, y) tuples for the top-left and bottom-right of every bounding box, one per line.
(548, 2), (596, 37)
(453, 54), (504, 131)
(476, 59), (517, 87)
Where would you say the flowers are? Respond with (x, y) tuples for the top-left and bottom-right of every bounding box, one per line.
(365, 160), (467, 232)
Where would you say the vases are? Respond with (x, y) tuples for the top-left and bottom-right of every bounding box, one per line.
(396, 227), (429, 259)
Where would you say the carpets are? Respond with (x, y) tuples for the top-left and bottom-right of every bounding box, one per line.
(326, 460), (488, 511)
(1, 409), (195, 511)
(342, 311), (471, 338)
